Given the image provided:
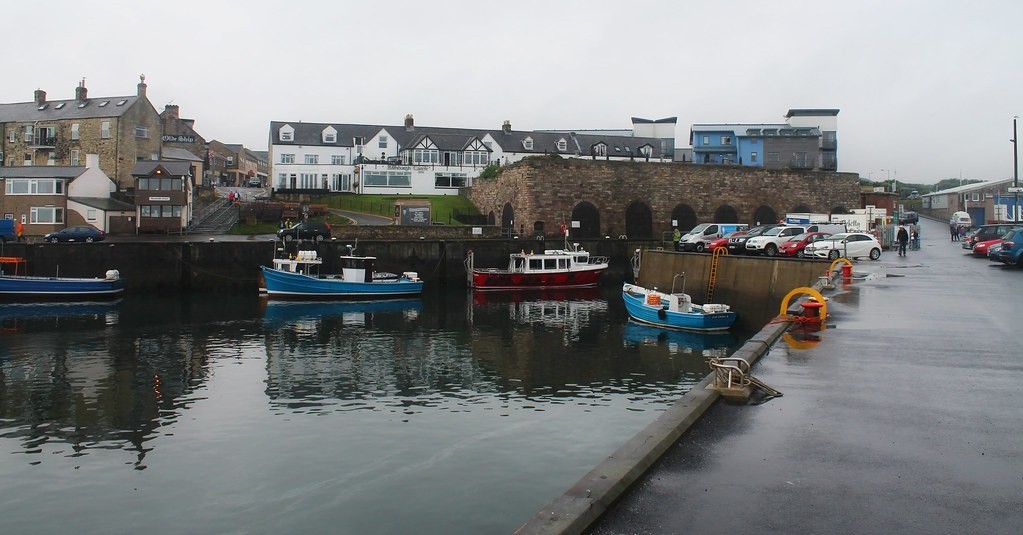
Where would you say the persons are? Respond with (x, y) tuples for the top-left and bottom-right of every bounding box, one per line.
(950, 222), (960, 241)
(228, 191), (234, 204)
(233, 190), (240, 201)
(960, 225), (966, 236)
(17, 222), (26, 242)
(285, 218), (292, 228)
(672, 228), (681, 252)
(896, 225), (908, 256)
(279, 221), (285, 229)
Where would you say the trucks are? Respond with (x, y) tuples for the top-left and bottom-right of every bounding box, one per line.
(0, 219), (16, 244)
(950, 211), (971, 230)
(678, 223), (748, 253)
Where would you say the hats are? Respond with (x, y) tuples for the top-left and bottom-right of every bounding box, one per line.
(898, 225), (904, 228)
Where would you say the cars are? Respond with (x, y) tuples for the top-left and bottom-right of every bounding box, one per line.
(709, 231), (747, 255)
(776, 232), (832, 259)
(44, 224), (107, 244)
(962, 222), (1023, 268)
(804, 233), (883, 261)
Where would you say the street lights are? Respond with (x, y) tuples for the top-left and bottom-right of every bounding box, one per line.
(881, 168), (890, 192)
(868, 172), (873, 186)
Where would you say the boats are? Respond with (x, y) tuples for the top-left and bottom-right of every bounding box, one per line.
(0, 257), (126, 302)
(260, 220), (425, 301)
(621, 280), (737, 336)
(463, 213), (611, 292)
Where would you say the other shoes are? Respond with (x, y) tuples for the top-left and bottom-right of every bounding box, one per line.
(904, 255), (906, 257)
(897, 252), (902, 257)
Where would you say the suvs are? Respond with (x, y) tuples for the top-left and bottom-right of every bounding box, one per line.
(249, 177), (262, 188)
(745, 225), (819, 256)
(898, 210), (919, 226)
(277, 222), (332, 241)
(727, 224), (778, 255)
